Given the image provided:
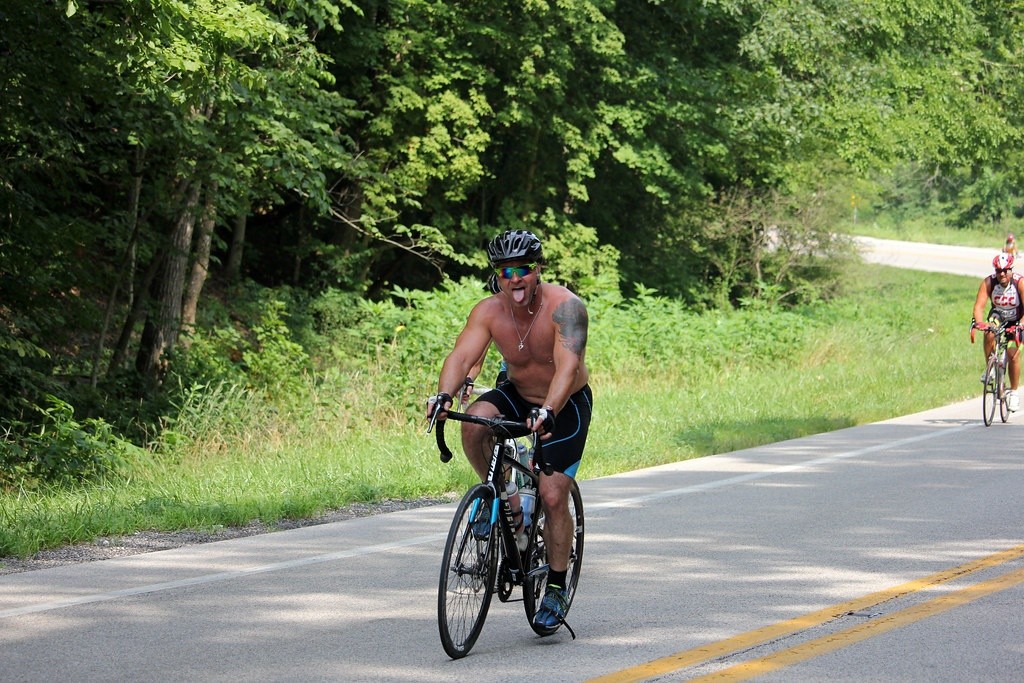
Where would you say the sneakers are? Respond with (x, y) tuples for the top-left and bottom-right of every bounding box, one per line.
(1008, 393), (1020, 412)
(980, 369), (995, 383)
(532, 583), (570, 630)
(469, 496), (493, 541)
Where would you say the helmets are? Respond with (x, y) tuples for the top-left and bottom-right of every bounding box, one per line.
(992, 253), (1015, 269)
(486, 228), (543, 268)
(1008, 234), (1014, 240)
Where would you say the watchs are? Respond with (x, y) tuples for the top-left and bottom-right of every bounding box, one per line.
(543, 404), (556, 418)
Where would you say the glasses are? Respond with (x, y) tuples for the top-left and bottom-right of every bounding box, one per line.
(994, 268), (1012, 274)
(493, 262), (538, 280)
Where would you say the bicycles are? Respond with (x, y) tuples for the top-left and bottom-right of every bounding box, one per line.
(424, 392), (586, 660)
(969, 317), (1024, 427)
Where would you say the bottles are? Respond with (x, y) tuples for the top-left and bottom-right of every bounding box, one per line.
(999, 363), (1005, 384)
(505, 480), (525, 534)
(518, 485), (536, 526)
(517, 444), (531, 484)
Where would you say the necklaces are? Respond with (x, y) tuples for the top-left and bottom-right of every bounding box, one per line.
(509, 304), (544, 351)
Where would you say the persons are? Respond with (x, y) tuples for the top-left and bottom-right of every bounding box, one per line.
(1002, 235), (1023, 271)
(424, 229), (592, 631)
(973, 253), (1024, 412)
(455, 335), (536, 536)
